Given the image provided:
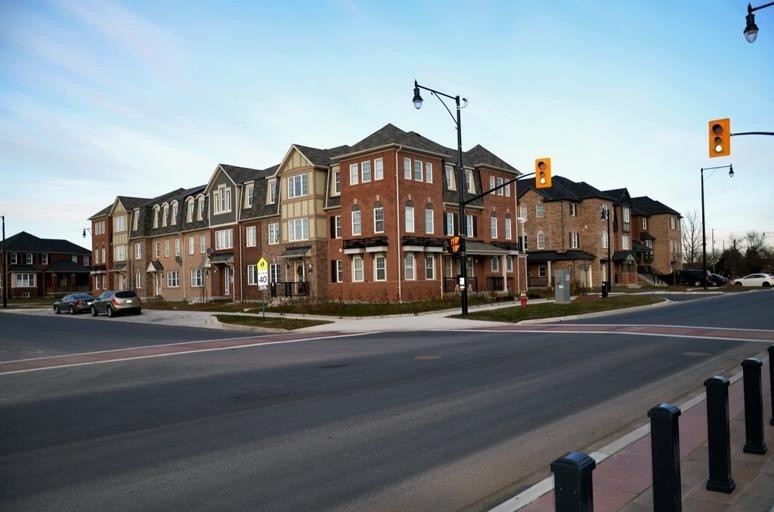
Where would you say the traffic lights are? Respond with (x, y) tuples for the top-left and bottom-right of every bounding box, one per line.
(708, 118), (730, 157)
(535, 158), (552, 189)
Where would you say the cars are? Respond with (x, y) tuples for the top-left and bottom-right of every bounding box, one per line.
(712, 274), (728, 286)
(734, 273), (774, 287)
(53, 294), (95, 314)
(91, 290), (142, 317)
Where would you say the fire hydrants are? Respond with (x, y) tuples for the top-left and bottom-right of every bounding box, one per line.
(521, 292), (528, 309)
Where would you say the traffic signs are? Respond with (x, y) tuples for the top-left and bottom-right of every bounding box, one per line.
(258, 273), (269, 286)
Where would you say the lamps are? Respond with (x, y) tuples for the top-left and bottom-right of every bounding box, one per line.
(83, 227), (92, 238)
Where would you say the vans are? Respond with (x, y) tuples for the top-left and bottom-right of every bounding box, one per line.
(672, 269), (711, 286)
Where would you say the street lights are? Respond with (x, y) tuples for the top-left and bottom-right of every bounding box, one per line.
(700, 164), (734, 289)
(412, 80), (468, 315)
(744, 2), (774, 43)
